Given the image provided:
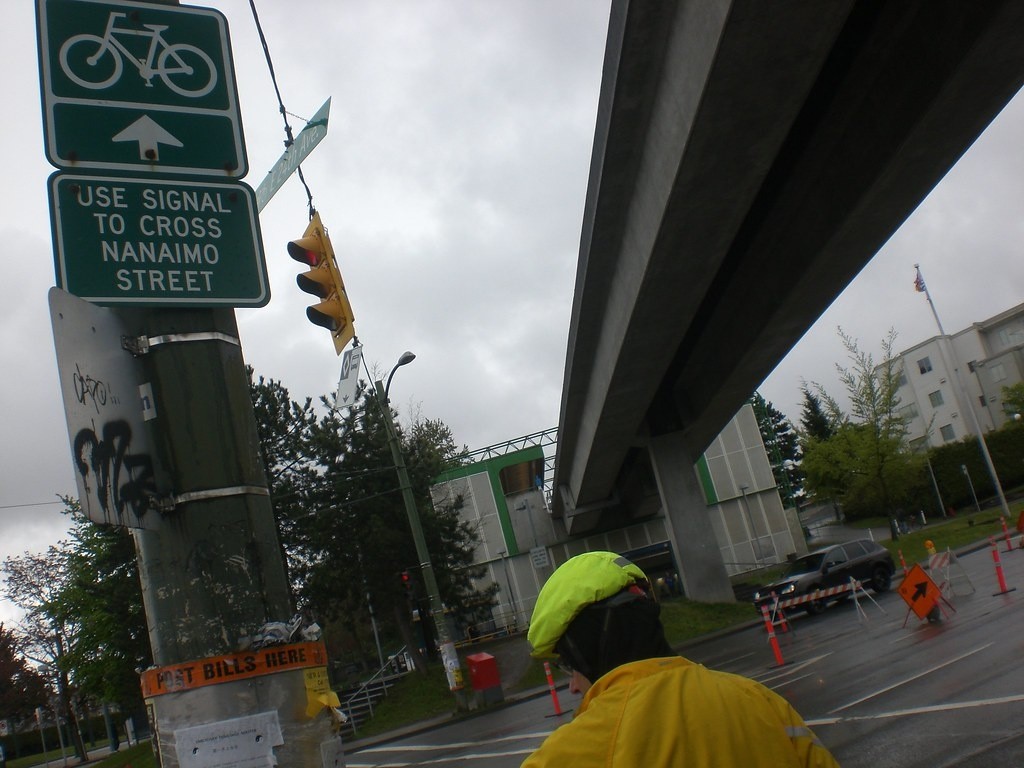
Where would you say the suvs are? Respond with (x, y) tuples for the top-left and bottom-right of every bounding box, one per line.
(755, 537), (897, 620)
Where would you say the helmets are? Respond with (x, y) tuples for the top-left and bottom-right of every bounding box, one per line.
(528, 550), (648, 658)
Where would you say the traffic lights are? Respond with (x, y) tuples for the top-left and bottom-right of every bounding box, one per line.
(401, 572), (417, 599)
(286, 210), (357, 357)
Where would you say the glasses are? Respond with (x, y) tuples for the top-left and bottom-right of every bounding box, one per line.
(551, 655), (572, 676)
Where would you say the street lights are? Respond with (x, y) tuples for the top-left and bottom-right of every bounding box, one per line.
(497, 548), (518, 615)
(738, 485), (767, 573)
(373, 351), (471, 714)
(512, 498), (549, 581)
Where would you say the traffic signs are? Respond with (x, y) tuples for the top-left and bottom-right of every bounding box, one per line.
(32, 0), (274, 310)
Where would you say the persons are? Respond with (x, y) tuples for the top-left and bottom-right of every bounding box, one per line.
(518, 551), (840, 768)
